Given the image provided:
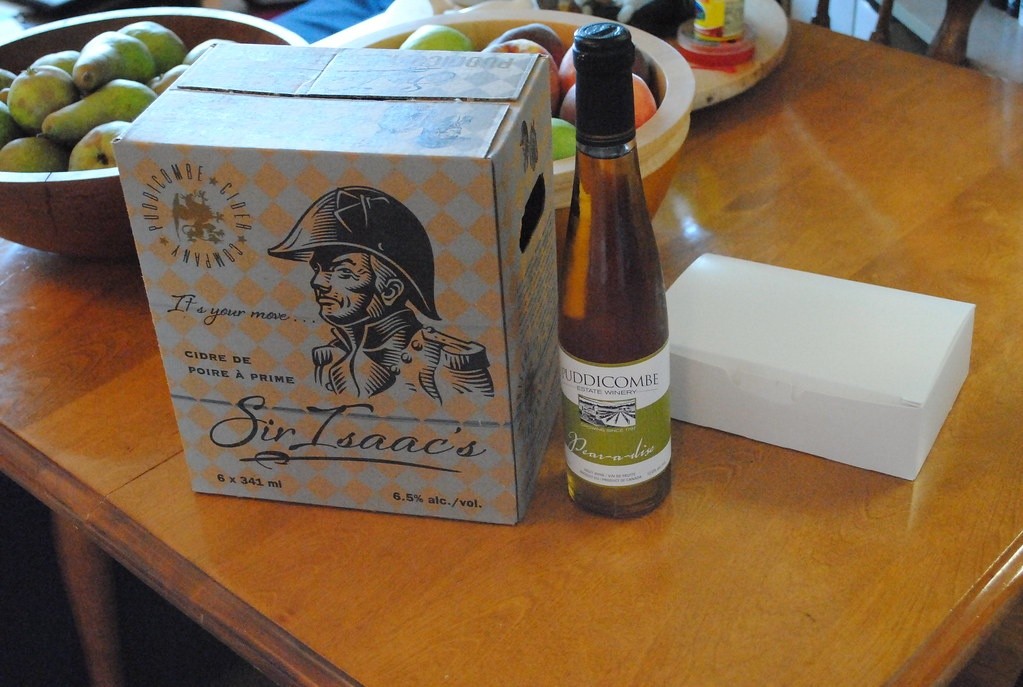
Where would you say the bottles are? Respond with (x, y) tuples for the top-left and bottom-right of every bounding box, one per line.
(553, 20), (672, 521)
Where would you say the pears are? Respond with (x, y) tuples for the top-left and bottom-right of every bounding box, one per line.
(0, 20), (238, 173)
(551, 116), (577, 160)
(399, 24), (475, 51)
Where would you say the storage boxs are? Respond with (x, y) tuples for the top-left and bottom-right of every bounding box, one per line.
(666, 253), (975, 482)
(111, 43), (561, 525)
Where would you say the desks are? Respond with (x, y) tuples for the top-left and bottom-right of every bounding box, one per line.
(0, 0), (1023, 687)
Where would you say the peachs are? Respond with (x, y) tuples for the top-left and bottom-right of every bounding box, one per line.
(454, 23), (659, 138)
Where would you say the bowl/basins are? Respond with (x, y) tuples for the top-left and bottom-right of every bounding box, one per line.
(0, 7), (311, 263)
(334, 6), (695, 254)
